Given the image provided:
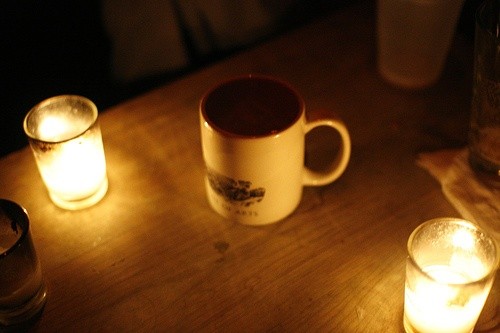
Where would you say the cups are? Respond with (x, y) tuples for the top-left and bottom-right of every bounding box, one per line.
(24, 95), (109, 212)
(0, 198), (47, 326)
(379, 0), (457, 89)
(401, 215), (500, 333)
(200, 77), (351, 225)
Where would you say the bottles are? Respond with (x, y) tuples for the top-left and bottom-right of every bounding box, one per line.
(470, 0), (500, 191)
